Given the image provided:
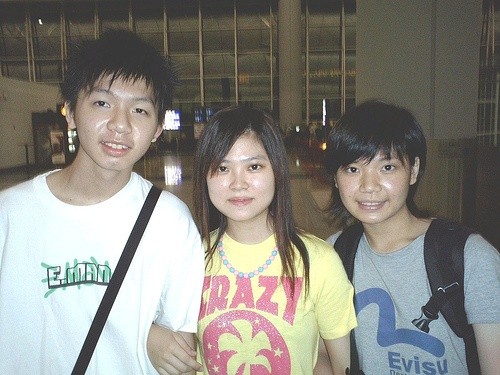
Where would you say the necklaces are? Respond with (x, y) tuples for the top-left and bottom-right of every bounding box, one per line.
(216, 225), (283, 279)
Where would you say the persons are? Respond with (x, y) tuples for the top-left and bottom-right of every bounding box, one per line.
(142, 102), (360, 375)
(0, 28), (204, 375)
(314, 96), (500, 375)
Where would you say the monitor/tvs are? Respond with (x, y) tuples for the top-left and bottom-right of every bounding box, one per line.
(163, 109), (181, 132)
